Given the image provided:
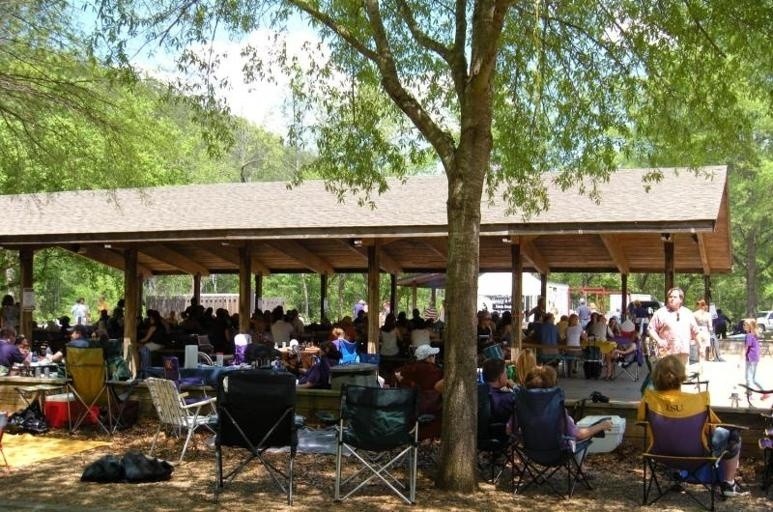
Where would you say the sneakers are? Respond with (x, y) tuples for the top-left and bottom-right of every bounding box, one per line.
(722, 479), (751, 496)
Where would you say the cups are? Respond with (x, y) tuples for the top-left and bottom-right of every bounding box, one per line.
(34, 367), (41, 378)
(31, 355), (36, 362)
(216, 354), (223, 366)
(44, 367), (49, 377)
(281, 341), (286, 349)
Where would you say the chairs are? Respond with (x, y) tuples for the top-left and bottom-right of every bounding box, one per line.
(610, 337), (642, 381)
(331, 382), (419, 506)
(161, 354), (212, 404)
(214, 369), (306, 506)
(337, 338), (361, 364)
(477, 383), (529, 484)
(65, 347), (130, 435)
(0, 412), (9, 467)
(513, 386), (610, 500)
(233, 333), (253, 369)
(635, 381), (725, 511)
(143, 376), (218, 467)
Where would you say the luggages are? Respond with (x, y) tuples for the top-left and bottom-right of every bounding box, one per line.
(583, 334), (602, 379)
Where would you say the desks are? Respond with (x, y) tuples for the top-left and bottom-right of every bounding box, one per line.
(29, 362), (57, 377)
(14, 385), (64, 417)
(278, 346), (320, 368)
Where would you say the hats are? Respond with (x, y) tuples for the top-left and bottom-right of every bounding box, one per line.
(414, 344), (441, 360)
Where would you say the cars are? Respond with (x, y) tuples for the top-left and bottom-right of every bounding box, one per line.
(742, 311), (773, 333)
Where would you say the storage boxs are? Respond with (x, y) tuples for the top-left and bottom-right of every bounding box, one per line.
(45, 392), (99, 428)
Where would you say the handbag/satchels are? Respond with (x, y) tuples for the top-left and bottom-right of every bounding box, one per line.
(338, 338), (360, 366)
(108, 356), (130, 381)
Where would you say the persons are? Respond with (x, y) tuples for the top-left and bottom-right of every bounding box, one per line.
(637, 353), (753, 496)
(646, 286), (732, 381)
(743, 318), (771, 400)
(0, 293), (644, 481)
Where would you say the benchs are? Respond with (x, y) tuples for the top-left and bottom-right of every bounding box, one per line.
(381, 355), (440, 366)
(150, 349), (184, 367)
(522, 343), (605, 378)
